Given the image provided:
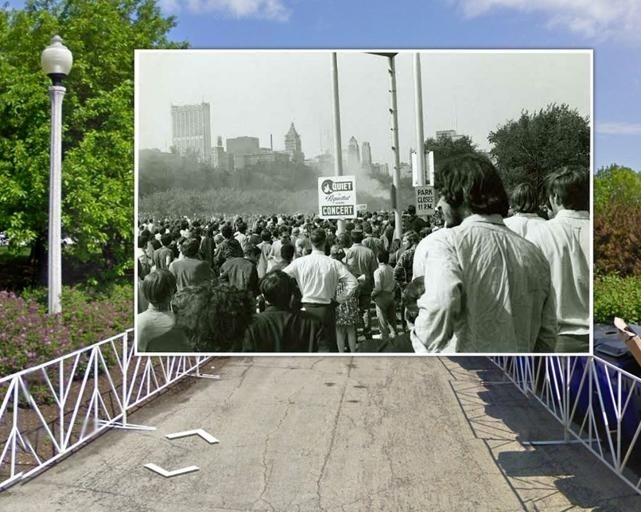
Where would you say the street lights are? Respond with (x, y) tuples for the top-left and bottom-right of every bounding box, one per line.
(41, 35), (74, 314)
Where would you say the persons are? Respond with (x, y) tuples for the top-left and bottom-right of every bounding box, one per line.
(546, 199), (556, 221)
(537, 197), (548, 218)
(412, 153), (557, 354)
(501, 176), (547, 243)
(523, 162), (589, 353)
